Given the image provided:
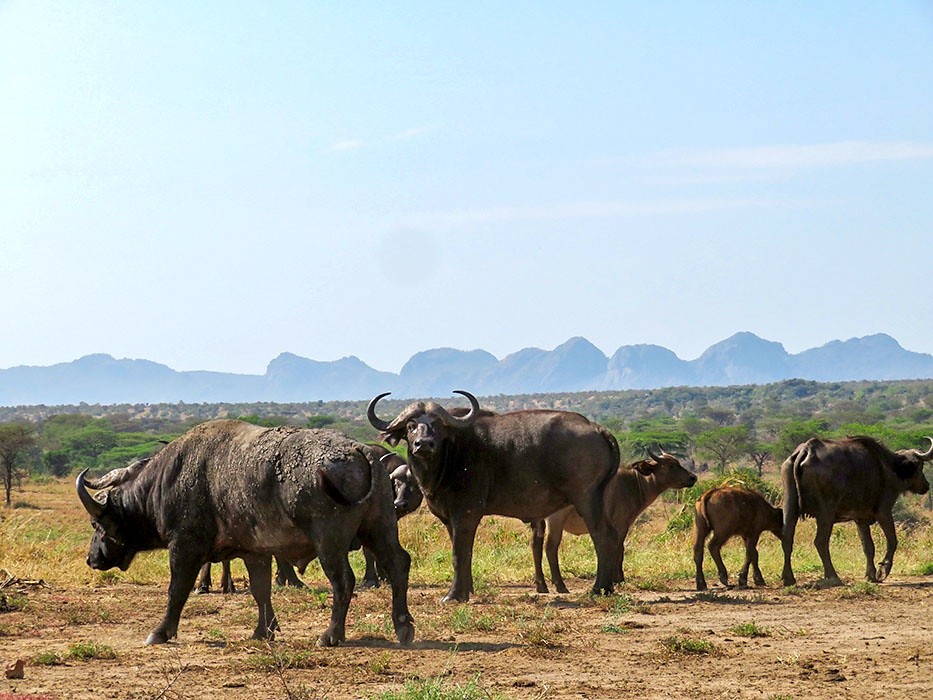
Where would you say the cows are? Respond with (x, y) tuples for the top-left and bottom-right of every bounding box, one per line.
(689, 481), (784, 596)
(367, 383), (624, 603)
(196, 441), (422, 594)
(71, 413), (418, 654)
(541, 439), (698, 605)
(776, 429), (932, 589)
